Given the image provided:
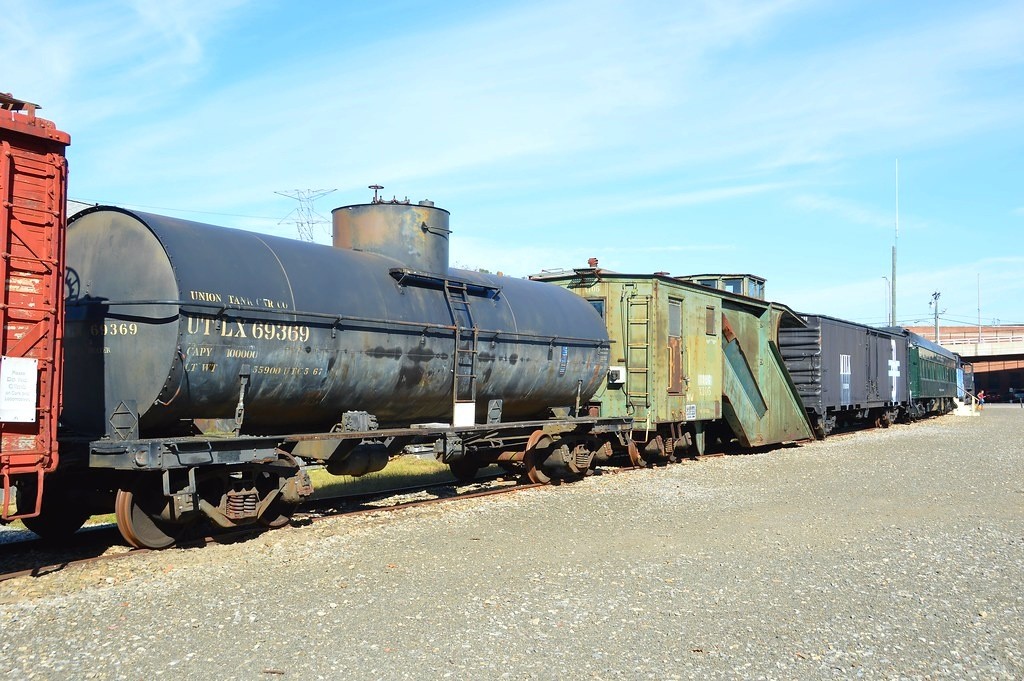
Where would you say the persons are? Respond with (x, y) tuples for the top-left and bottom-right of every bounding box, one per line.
(976, 390), (986, 410)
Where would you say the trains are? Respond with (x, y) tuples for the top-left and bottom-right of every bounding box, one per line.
(1, 90), (974, 552)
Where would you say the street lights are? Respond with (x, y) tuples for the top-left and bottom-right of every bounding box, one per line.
(914, 320), (939, 344)
(882, 276), (890, 327)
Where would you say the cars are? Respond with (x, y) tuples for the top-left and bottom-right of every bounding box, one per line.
(986, 391), (1001, 402)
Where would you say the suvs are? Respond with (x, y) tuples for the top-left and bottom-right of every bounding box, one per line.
(1010, 388), (1024, 402)
